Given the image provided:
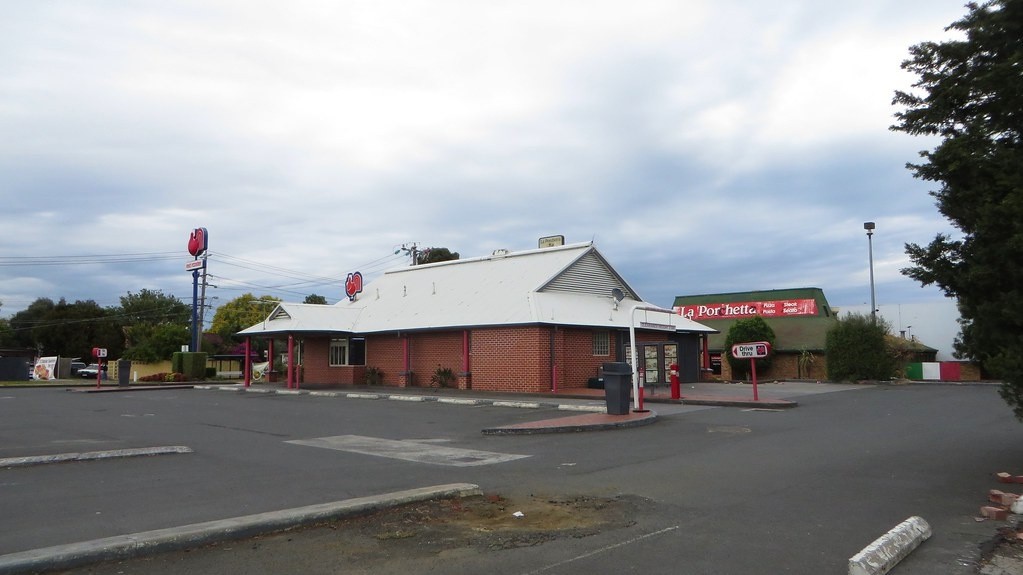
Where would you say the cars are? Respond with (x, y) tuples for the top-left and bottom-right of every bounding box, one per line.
(72, 362), (86, 369)
(77, 363), (106, 378)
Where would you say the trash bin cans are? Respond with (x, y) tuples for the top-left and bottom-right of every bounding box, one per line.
(602, 362), (632, 415)
